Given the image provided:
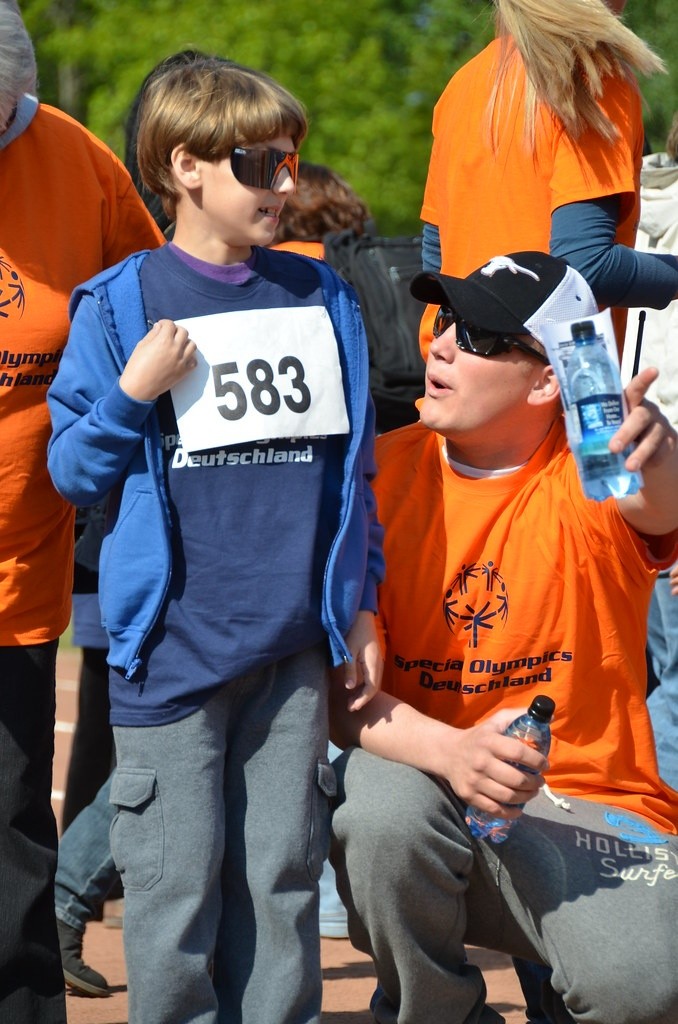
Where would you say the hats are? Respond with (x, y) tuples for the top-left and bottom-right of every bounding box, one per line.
(410, 251), (599, 355)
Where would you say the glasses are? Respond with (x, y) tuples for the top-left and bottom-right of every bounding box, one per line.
(433, 305), (550, 367)
(190, 146), (300, 191)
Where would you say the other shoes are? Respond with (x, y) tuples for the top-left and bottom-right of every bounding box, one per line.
(56, 918), (109, 995)
(478, 1005), (505, 1024)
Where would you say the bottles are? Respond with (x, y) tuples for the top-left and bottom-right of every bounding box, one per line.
(465, 694), (555, 843)
(568, 321), (640, 501)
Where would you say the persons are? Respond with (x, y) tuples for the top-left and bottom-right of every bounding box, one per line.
(46, 56), (387, 1024)
(0, 0), (678, 1024)
